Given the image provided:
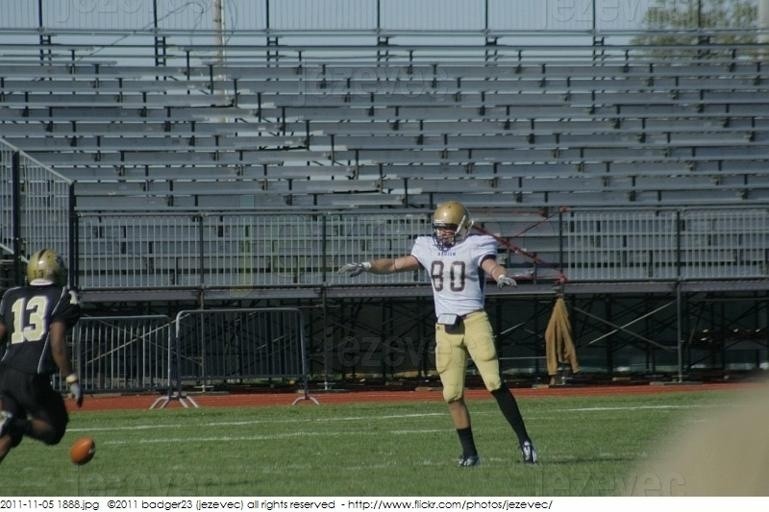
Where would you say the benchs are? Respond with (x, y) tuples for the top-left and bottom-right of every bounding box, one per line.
(0, 28), (769, 286)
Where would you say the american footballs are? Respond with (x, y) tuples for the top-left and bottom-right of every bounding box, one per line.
(70, 437), (95, 465)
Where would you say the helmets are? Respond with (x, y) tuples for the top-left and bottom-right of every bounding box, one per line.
(431, 200), (469, 228)
(24, 248), (68, 286)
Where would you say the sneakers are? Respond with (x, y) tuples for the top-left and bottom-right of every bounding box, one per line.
(459, 454), (479, 466)
(521, 440), (536, 464)
(0, 410), (13, 438)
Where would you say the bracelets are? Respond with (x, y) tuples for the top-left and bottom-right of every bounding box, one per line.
(66, 373), (78, 384)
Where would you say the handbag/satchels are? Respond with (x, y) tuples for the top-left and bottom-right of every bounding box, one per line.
(437, 313), (465, 334)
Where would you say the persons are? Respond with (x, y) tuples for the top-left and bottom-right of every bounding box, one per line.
(0, 250), (85, 462)
(337, 201), (540, 467)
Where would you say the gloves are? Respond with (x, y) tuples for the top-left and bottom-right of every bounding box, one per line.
(496, 273), (517, 290)
(338, 262), (371, 277)
(66, 373), (83, 408)
